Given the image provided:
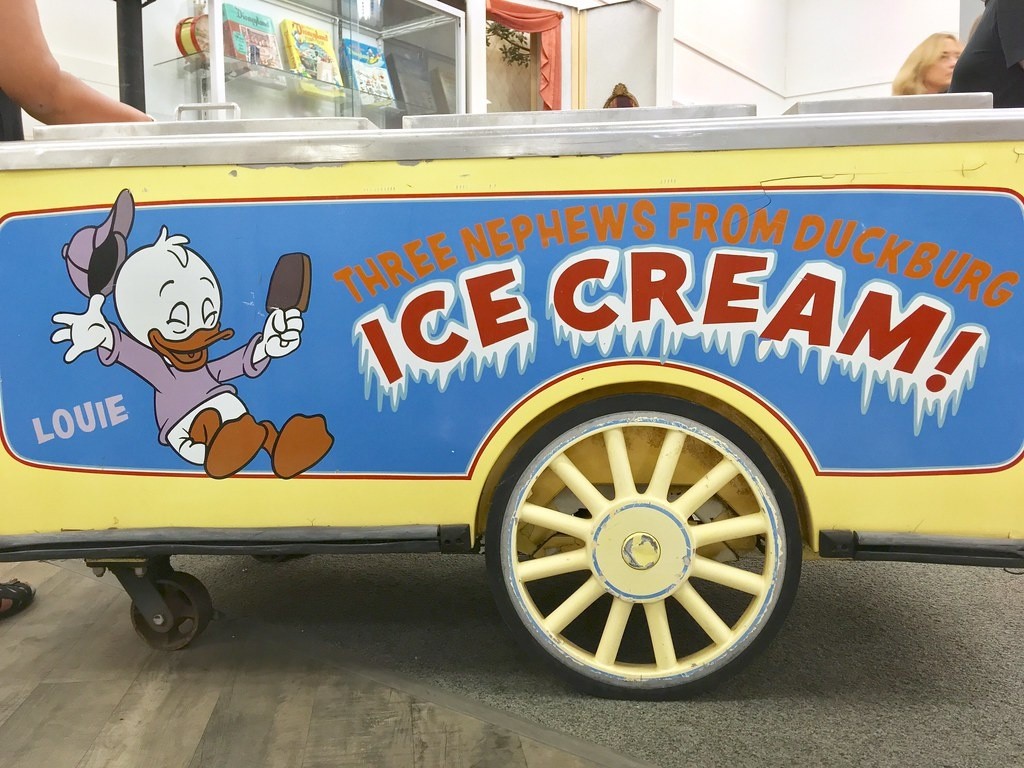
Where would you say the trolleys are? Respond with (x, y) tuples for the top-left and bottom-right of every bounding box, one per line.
(0, 91), (1024, 696)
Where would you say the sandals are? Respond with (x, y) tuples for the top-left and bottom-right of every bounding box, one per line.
(0, 580), (36, 617)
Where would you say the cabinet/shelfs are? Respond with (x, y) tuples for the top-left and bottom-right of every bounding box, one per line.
(141, 0), (467, 124)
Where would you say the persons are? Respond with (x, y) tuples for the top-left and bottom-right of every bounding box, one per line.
(951, 0), (1024, 110)
(0, 0), (161, 632)
(892, 31), (962, 96)
